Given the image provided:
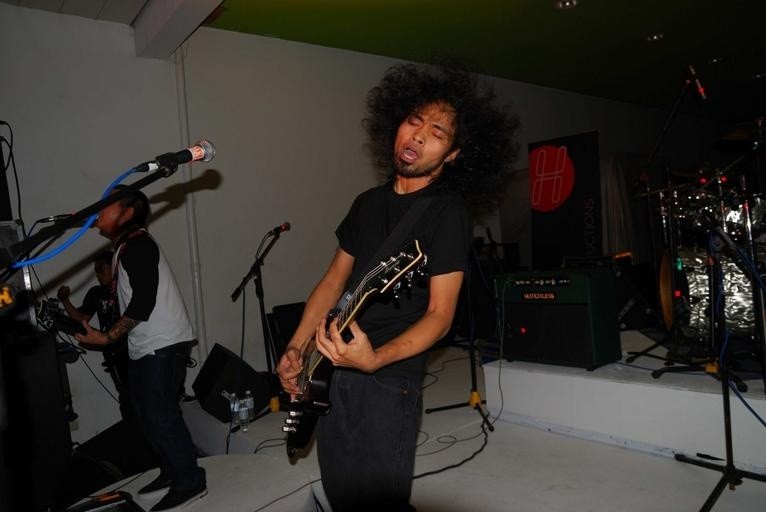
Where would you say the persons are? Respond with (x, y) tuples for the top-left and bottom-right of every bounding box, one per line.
(268, 61), (472, 512)
(71, 181), (210, 511)
(56, 250), (137, 470)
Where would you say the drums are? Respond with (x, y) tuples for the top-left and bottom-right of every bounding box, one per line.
(661, 245), (757, 341)
(722, 194), (766, 245)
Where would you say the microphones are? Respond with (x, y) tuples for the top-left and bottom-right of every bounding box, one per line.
(54, 214), (96, 228)
(156, 138), (215, 167)
(703, 210), (736, 250)
(274, 222), (290, 233)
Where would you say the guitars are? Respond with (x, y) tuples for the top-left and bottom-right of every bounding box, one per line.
(282, 241), (427, 465)
(35, 296), (128, 392)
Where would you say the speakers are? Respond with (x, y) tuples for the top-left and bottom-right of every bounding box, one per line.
(450, 242), (520, 344)
(501, 267), (622, 371)
(192, 301), (306, 423)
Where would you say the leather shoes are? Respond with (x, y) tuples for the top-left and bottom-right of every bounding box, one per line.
(150, 490), (208, 512)
(139, 476), (171, 493)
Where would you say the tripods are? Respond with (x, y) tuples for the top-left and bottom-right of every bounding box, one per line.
(424, 247), (495, 432)
(672, 254), (766, 512)
(230, 271), (292, 433)
(625, 190), (698, 365)
(652, 197), (748, 392)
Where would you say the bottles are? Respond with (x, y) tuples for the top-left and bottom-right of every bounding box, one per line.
(228, 389), (256, 431)
